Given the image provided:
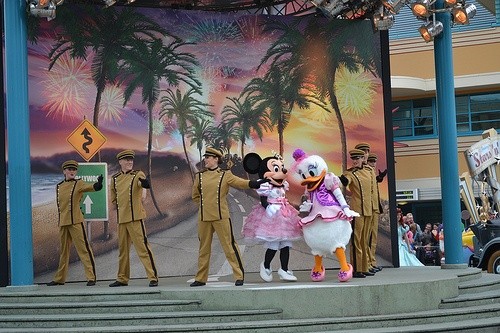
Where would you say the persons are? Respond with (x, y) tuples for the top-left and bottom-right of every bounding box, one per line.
(396, 208), (497, 267)
(108, 150), (159, 287)
(189, 146), (271, 286)
(338, 143), (387, 278)
(46, 160), (104, 286)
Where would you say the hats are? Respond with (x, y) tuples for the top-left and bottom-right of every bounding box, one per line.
(348, 148), (365, 158)
(355, 141), (370, 149)
(62, 160), (78, 170)
(203, 147), (223, 157)
(116, 149), (135, 160)
(367, 152), (377, 161)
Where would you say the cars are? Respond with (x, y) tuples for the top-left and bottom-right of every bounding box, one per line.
(470, 220), (500, 274)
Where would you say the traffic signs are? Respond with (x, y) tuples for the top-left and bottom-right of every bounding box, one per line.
(74, 162), (108, 222)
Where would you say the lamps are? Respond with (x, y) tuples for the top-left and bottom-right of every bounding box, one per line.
(26, 0), (64, 21)
(311, 0), (349, 19)
(95, 0), (116, 9)
(370, 0), (477, 43)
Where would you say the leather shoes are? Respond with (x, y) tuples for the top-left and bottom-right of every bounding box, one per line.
(109, 281), (129, 287)
(190, 281), (205, 287)
(47, 281), (64, 286)
(354, 265), (383, 278)
(149, 280), (158, 286)
(87, 280), (95, 286)
(235, 279), (243, 286)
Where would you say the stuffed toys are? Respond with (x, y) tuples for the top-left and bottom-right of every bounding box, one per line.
(289, 149), (361, 282)
(241, 153), (304, 282)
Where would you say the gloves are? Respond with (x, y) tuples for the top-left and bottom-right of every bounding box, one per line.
(376, 168), (387, 180)
(339, 175), (348, 186)
(249, 179), (260, 189)
(138, 174), (151, 189)
(94, 183), (103, 191)
